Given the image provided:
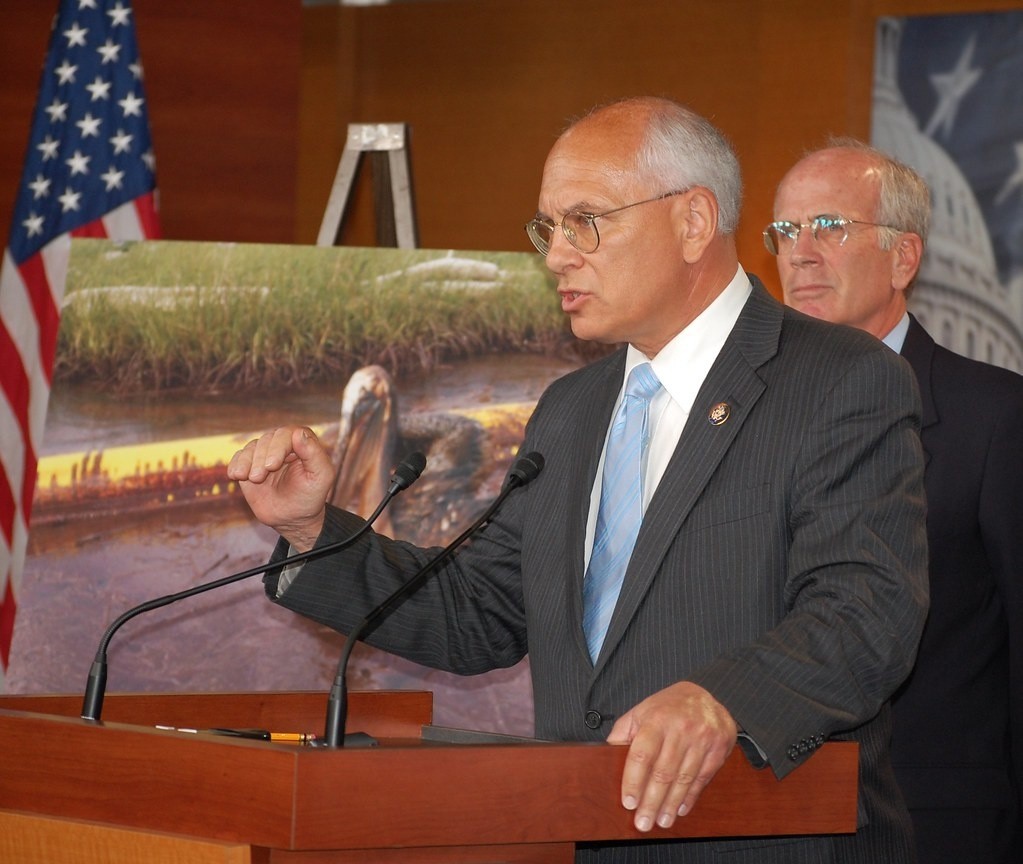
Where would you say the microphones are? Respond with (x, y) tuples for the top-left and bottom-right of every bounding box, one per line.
(324, 449), (544, 749)
(77, 449), (427, 722)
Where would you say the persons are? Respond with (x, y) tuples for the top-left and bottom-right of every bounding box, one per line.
(766, 143), (1023, 864)
(228, 96), (929, 864)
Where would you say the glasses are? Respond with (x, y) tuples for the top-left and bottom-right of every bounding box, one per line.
(760, 212), (903, 256)
(525, 190), (688, 257)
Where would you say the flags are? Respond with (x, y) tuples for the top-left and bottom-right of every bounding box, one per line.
(1, 0), (159, 669)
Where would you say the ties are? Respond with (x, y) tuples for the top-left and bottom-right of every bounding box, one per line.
(583, 363), (662, 668)
